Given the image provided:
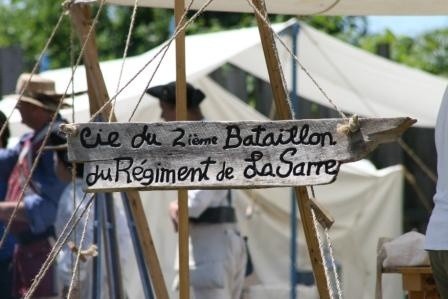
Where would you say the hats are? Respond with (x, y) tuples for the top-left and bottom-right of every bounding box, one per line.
(146, 81), (205, 104)
(4, 73), (58, 113)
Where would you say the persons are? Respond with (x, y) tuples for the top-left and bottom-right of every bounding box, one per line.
(422, 85), (448, 299)
(0, 73), (95, 299)
(147, 81), (248, 299)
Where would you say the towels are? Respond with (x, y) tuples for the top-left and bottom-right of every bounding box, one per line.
(380, 230), (431, 268)
